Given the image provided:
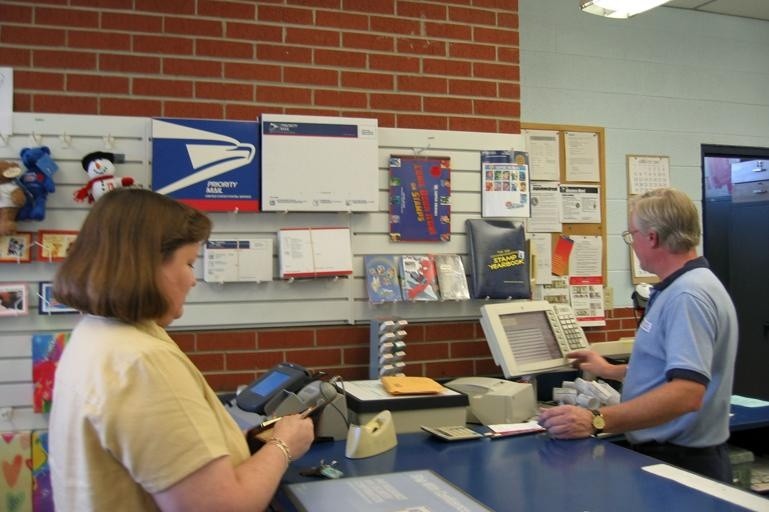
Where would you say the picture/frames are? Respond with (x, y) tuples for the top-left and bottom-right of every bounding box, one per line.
(0, 230), (79, 318)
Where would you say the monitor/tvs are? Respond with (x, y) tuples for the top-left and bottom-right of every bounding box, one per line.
(480, 299), (576, 379)
(283, 466), (496, 512)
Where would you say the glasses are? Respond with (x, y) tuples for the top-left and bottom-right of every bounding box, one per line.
(621, 230), (639, 245)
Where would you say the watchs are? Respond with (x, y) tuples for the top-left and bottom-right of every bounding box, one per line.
(591, 410), (606, 436)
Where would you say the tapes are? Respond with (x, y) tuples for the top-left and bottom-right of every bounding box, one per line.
(365, 422), (379, 433)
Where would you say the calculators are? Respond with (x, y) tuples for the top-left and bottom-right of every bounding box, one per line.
(420, 424), (483, 442)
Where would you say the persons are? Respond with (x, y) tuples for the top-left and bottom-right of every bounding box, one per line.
(537, 187), (741, 484)
(47, 189), (316, 512)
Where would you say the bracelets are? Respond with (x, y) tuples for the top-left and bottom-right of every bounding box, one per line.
(268, 437), (291, 467)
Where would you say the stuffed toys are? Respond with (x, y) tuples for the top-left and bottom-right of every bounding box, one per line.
(0, 160), (27, 236)
(72, 151), (134, 202)
(14, 146), (56, 222)
